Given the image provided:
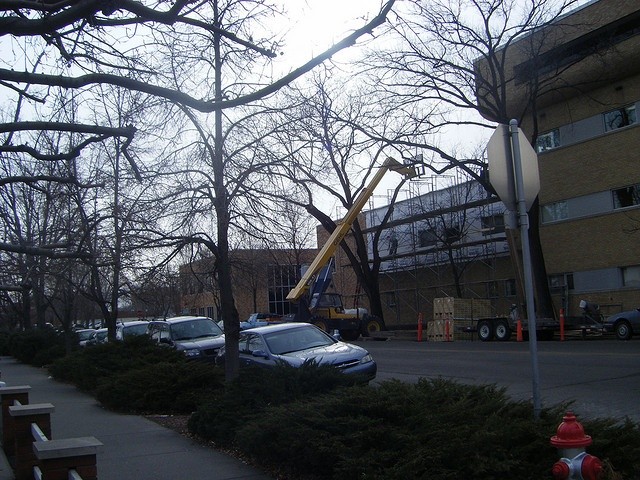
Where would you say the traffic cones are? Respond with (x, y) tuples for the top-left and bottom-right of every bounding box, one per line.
(516, 319), (524, 343)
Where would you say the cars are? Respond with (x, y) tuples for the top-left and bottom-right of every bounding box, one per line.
(86, 328), (108, 345)
(75, 328), (96, 347)
(604, 309), (640, 340)
(147, 315), (225, 362)
(218, 323), (377, 387)
(104, 319), (149, 340)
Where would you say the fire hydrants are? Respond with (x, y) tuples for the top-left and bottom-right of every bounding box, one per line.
(551, 412), (603, 480)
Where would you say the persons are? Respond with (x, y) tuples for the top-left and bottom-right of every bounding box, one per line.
(385, 234), (399, 256)
(508, 303), (524, 332)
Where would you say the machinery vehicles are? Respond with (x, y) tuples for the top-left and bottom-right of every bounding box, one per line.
(286, 154), (425, 339)
(455, 303), (559, 341)
